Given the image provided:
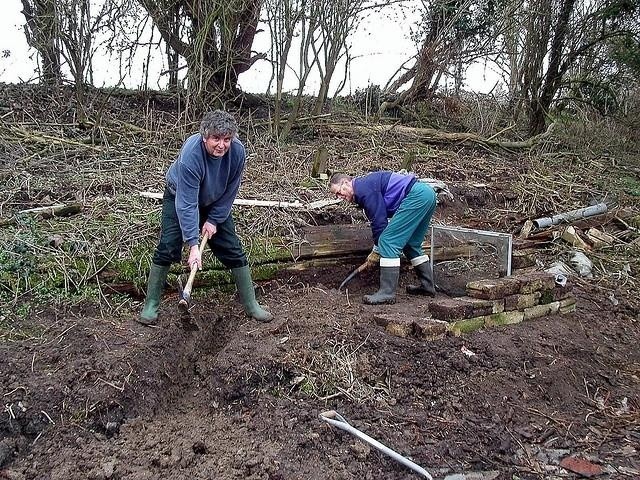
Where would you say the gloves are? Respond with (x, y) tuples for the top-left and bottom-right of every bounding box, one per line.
(364, 251), (380, 271)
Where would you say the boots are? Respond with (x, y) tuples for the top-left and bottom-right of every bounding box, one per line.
(140, 261), (173, 321)
(406, 255), (436, 297)
(231, 264), (274, 322)
(363, 256), (400, 305)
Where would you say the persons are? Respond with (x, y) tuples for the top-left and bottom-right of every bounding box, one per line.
(135, 108), (276, 326)
(326, 169), (441, 306)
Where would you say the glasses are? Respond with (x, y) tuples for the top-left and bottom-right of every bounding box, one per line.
(333, 182), (343, 200)
